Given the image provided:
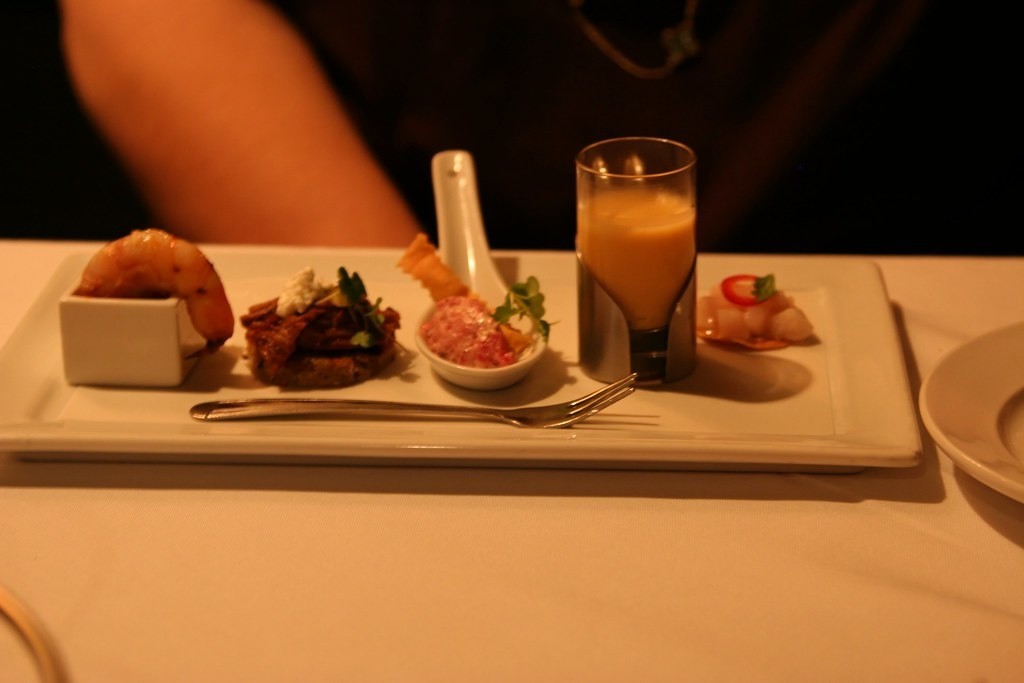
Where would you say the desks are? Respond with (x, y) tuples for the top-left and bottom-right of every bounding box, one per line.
(0, 239), (1024, 682)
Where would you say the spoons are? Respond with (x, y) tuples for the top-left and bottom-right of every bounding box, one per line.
(413, 149), (547, 390)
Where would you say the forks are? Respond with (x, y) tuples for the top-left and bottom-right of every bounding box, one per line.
(189, 374), (639, 428)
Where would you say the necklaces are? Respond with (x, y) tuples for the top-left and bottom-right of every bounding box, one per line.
(571, 0), (699, 78)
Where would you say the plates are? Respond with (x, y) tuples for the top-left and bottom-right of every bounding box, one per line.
(0, 243), (922, 471)
(918, 319), (1024, 504)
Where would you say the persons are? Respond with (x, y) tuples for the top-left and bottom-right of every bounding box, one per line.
(59, 0), (1024, 261)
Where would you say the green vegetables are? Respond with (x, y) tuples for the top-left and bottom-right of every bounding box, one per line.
(336, 266), (411, 355)
(751, 273), (777, 301)
(491, 274), (561, 338)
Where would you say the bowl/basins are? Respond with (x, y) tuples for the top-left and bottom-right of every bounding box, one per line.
(56, 278), (202, 390)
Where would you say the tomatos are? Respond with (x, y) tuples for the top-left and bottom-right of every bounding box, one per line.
(720, 275), (771, 306)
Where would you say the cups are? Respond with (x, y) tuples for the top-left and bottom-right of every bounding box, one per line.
(575, 138), (700, 386)
